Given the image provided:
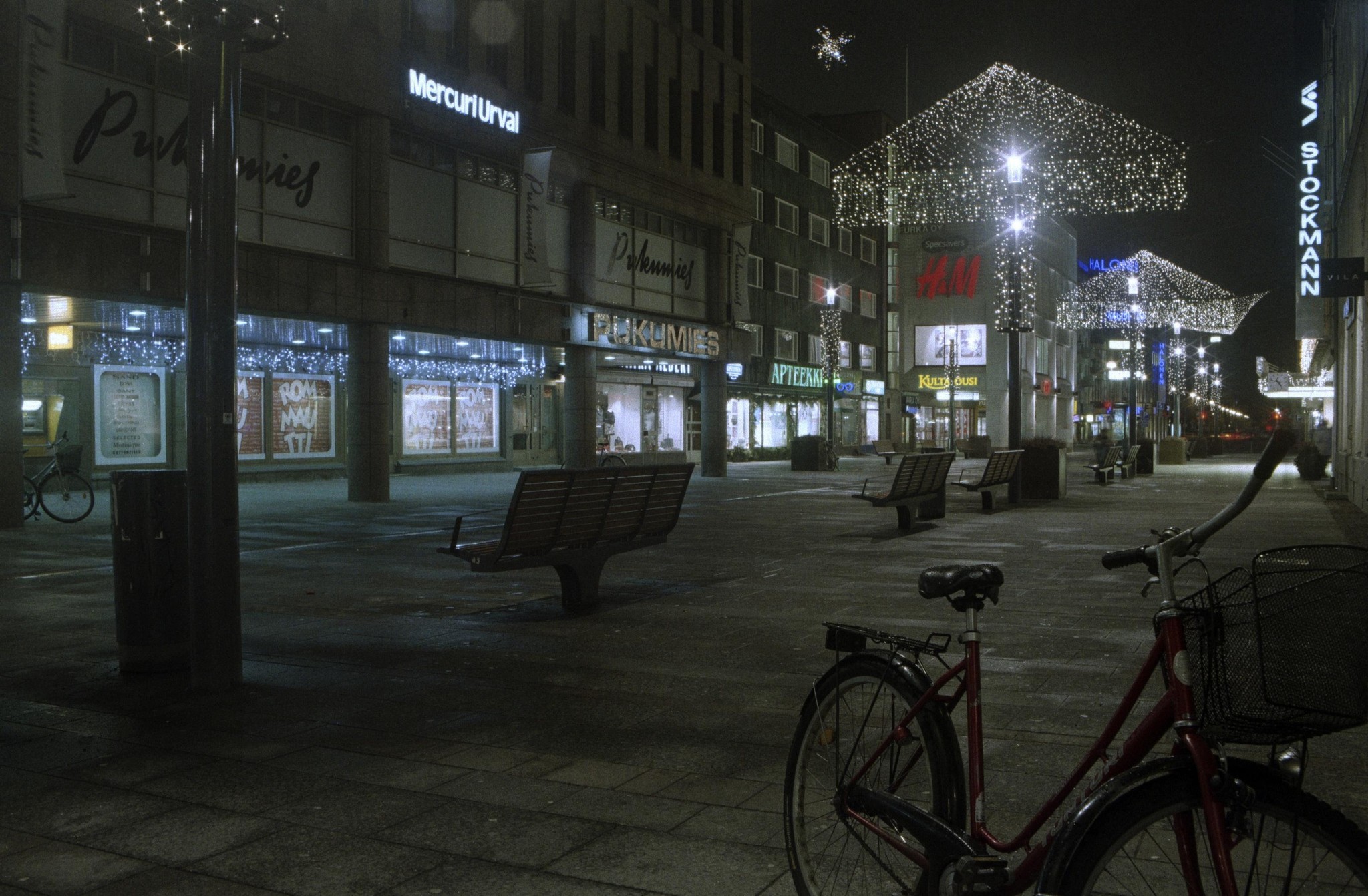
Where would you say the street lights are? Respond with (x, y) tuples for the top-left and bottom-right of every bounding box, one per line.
(947, 324), (958, 452)
(1002, 146), (1026, 500)
(1212, 363), (1220, 454)
(1197, 345), (1206, 457)
(1172, 317), (1181, 437)
(1126, 275), (1141, 475)
(825, 287), (837, 449)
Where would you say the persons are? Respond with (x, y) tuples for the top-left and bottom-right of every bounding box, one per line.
(1189, 419), (1208, 457)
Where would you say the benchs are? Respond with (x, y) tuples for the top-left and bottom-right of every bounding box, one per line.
(1186, 441), (1197, 461)
(1083, 445), (1141, 483)
(852, 439), (1025, 532)
(436, 461), (696, 611)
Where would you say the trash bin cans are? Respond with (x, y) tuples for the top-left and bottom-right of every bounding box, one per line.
(109, 470), (190, 673)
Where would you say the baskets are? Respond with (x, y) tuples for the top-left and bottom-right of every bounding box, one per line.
(1176, 544), (1368, 748)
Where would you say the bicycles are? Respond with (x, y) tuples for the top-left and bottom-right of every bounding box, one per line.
(560, 440), (627, 470)
(23, 430), (95, 524)
(777, 416), (1368, 896)
(825, 440), (839, 472)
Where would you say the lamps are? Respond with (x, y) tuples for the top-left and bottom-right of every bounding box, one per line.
(1073, 390), (1080, 398)
(1034, 385), (1042, 393)
(1054, 388), (1062, 395)
